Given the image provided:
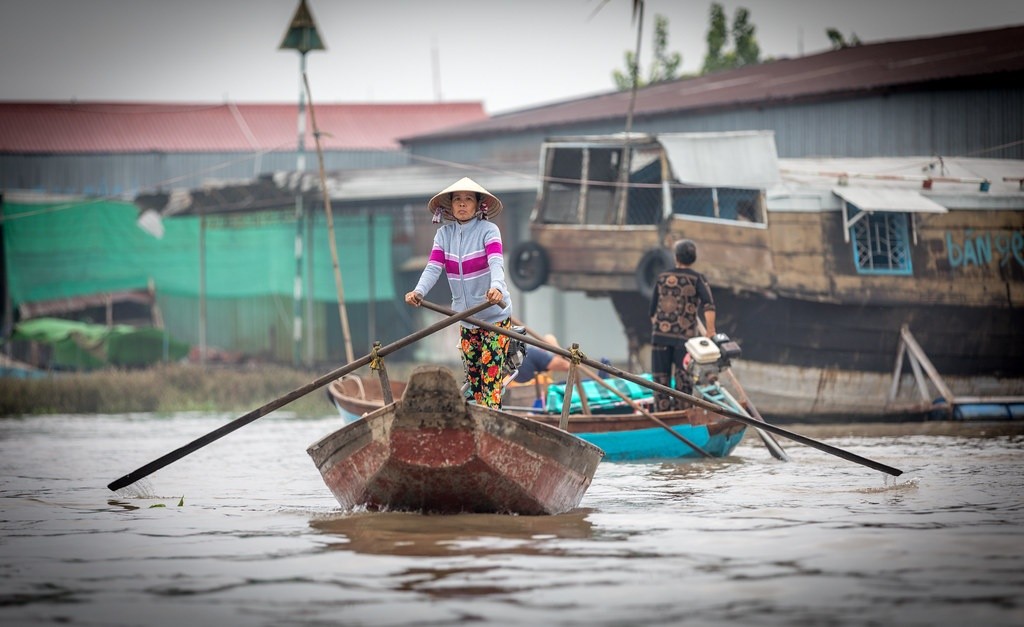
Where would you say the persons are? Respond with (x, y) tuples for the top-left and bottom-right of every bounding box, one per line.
(648, 239), (716, 413)
(508, 334), (571, 388)
(404, 177), (513, 412)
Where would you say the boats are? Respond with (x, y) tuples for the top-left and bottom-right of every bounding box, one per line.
(510, 131), (1024, 420)
(308, 333), (748, 516)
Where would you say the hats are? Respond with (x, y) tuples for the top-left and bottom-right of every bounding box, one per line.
(735, 199), (756, 222)
(428, 176), (504, 222)
